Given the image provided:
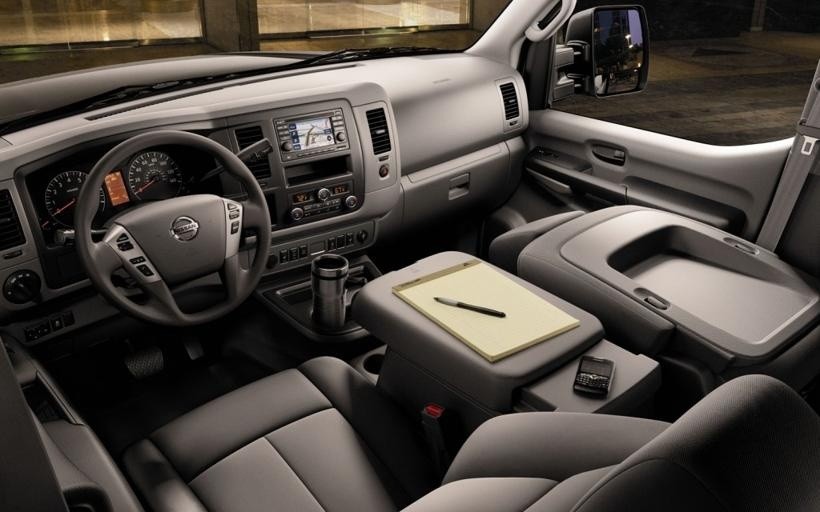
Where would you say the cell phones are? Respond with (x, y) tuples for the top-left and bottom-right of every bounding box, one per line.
(573, 355), (616, 400)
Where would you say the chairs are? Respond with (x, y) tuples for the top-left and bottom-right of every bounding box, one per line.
(124, 354), (819, 512)
(488, 204), (820, 375)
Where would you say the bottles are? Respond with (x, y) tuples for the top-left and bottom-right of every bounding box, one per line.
(312, 253), (349, 331)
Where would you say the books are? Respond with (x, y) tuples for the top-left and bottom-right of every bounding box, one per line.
(391, 259), (580, 362)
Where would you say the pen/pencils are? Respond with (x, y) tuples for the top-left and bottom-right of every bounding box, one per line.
(434, 297), (505, 318)
